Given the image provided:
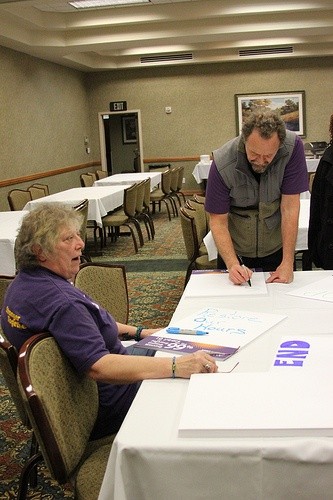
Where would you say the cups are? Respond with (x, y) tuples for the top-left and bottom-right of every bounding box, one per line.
(200, 154), (210, 164)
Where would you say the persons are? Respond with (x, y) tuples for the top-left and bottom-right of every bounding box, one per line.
(203, 110), (309, 285)
(0, 202), (218, 433)
(307, 114), (333, 270)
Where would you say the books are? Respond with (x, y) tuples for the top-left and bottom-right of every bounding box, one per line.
(132, 305), (290, 360)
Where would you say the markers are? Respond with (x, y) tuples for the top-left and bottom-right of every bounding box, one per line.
(166, 327), (209, 335)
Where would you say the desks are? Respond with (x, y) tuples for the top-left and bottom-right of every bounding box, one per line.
(203, 197), (312, 270)
(93, 171), (161, 213)
(99, 272), (333, 500)
(22, 184), (131, 254)
(0, 210), (27, 276)
(193, 157), (321, 193)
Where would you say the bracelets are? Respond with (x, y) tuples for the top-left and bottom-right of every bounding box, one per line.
(172, 357), (175, 378)
(135, 327), (143, 341)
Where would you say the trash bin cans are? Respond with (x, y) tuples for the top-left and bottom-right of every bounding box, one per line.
(149, 164), (171, 172)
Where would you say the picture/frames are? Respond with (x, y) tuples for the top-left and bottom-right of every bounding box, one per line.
(234, 90), (306, 139)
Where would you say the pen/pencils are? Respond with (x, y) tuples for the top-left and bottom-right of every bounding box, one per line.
(236, 253), (251, 286)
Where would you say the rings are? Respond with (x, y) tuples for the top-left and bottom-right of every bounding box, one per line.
(204, 364), (211, 370)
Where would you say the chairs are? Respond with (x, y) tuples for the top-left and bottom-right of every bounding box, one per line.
(0, 333), (37, 489)
(192, 194), (207, 205)
(186, 199), (207, 255)
(179, 206), (217, 287)
(69, 263), (129, 327)
(8, 165), (184, 255)
(17, 330), (116, 500)
